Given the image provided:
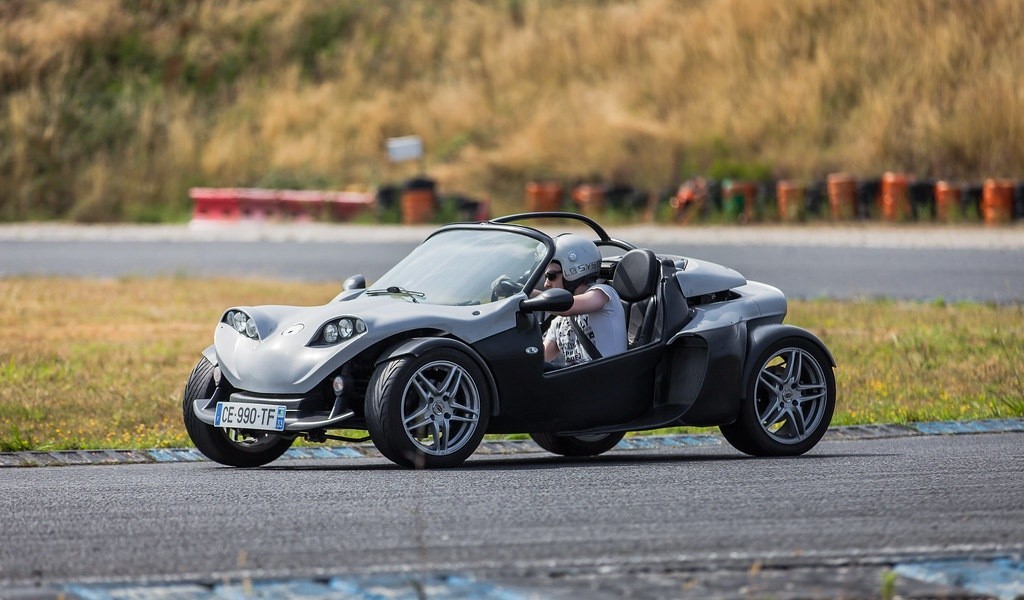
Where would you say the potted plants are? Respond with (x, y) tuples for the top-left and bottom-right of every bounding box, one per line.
(398, 174), (438, 225)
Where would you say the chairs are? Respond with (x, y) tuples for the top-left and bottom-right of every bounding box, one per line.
(529, 259), (546, 292)
(611, 247), (659, 350)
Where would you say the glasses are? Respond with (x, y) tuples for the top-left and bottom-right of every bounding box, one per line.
(544, 270), (562, 281)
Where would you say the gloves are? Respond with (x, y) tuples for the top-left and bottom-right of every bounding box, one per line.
(491, 275), (523, 296)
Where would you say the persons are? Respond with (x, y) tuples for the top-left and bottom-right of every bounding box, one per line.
(490, 233), (626, 368)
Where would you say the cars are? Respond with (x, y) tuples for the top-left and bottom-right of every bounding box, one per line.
(181, 210), (838, 472)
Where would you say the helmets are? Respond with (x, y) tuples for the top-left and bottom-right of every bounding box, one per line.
(535, 233), (602, 281)
